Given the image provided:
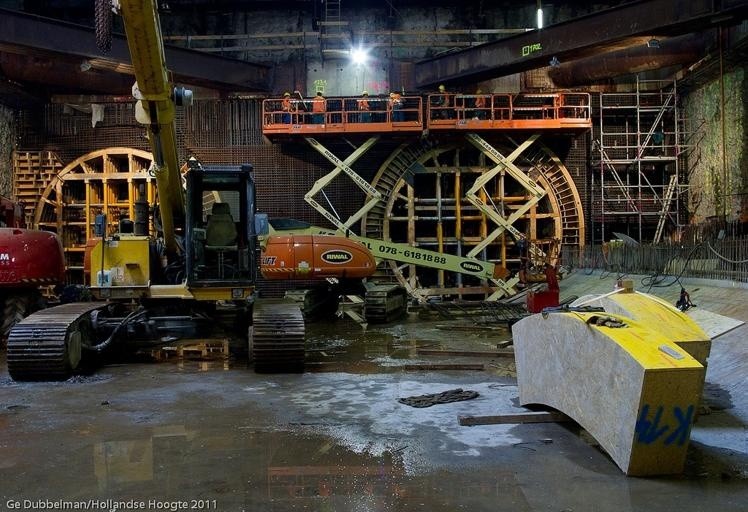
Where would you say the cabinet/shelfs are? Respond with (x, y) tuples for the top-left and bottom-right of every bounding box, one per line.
(32, 146), (166, 304)
(383, 145), (564, 304)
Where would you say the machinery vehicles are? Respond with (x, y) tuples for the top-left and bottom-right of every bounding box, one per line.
(5, 0), (309, 380)
(256, 214), (515, 324)
(0, 193), (67, 345)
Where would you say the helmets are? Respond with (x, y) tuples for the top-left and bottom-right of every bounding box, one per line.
(362, 91), (368, 96)
(439, 84), (445, 90)
(284, 92), (290, 96)
(476, 89), (482, 94)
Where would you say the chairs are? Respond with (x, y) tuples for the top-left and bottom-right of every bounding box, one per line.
(203, 202), (238, 280)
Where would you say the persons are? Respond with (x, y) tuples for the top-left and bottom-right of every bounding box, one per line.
(553, 92), (565, 118)
(475, 89), (486, 119)
(358, 92), (372, 123)
(437, 85), (449, 119)
(390, 91), (404, 121)
(281, 91), (292, 124)
(311, 92), (327, 124)
